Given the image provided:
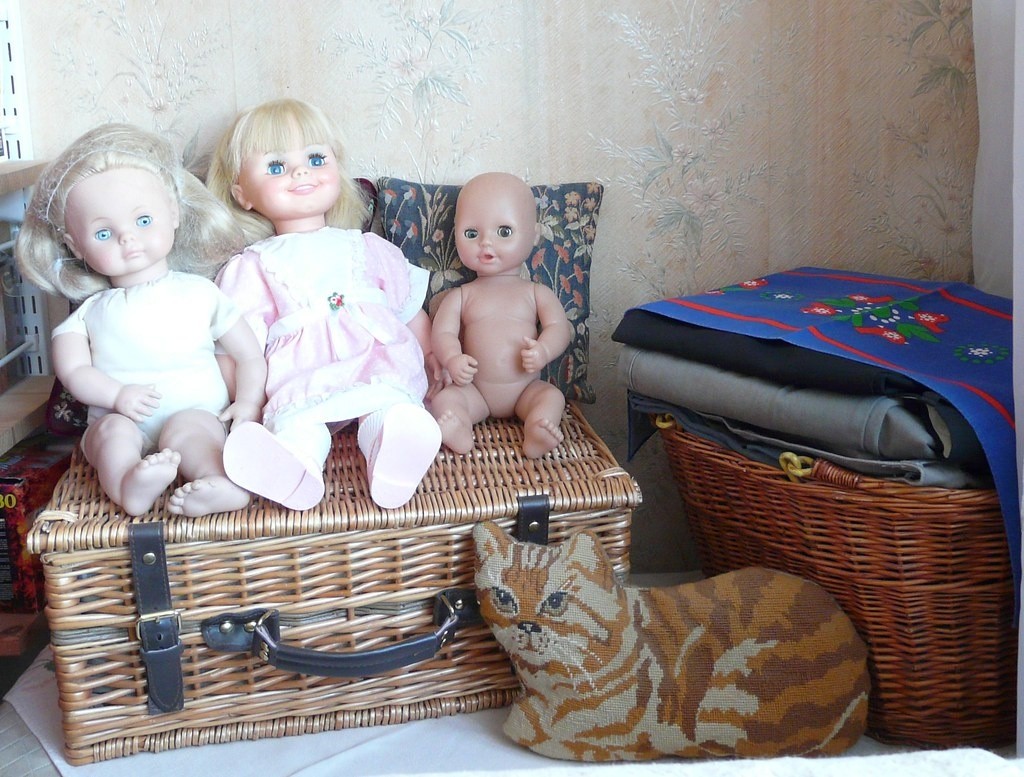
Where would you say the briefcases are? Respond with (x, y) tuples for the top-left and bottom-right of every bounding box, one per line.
(23, 393), (643, 768)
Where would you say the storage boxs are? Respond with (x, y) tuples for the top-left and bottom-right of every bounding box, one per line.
(0, 432), (72, 613)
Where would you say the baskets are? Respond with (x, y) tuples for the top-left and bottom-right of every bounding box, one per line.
(652, 413), (1017, 754)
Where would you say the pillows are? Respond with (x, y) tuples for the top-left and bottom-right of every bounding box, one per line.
(377, 175), (598, 403)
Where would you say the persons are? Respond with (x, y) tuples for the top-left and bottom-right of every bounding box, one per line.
(15, 123), (248, 517)
(431, 171), (571, 459)
(205, 98), (444, 510)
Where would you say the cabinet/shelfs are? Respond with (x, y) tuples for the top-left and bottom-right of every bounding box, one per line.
(0, 0), (72, 658)
